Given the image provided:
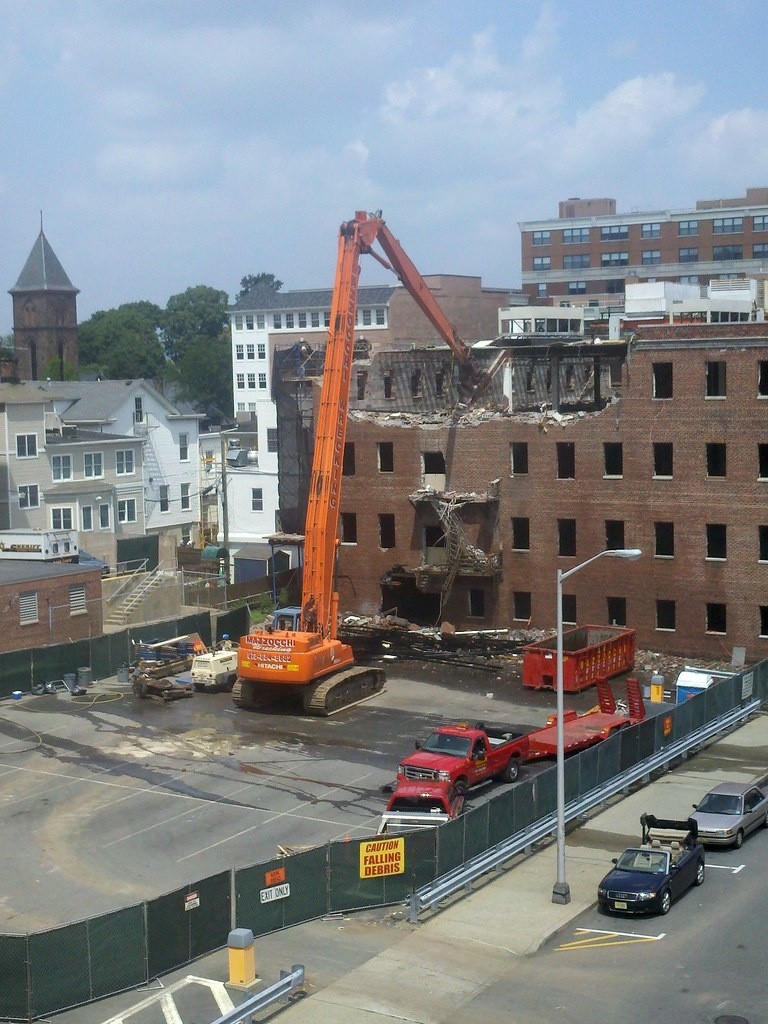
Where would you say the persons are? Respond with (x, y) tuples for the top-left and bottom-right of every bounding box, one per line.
(737, 802), (750, 811)
(660, 850), (676, 868)
(223, 634), (232, 651)
(186, 541), (195, 548)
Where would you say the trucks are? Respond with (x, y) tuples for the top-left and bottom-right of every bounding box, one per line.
(190, 650), (238, 692)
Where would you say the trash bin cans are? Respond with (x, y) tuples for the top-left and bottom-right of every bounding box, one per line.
(118, 662), (130, 682)
(63, 673), (76, 690)
(77, 666), (91, 687)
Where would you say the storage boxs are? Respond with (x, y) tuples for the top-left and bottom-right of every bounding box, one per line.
(12, 691), (22, 699)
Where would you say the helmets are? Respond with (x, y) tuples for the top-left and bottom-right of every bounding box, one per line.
(222, 633), (230, 640)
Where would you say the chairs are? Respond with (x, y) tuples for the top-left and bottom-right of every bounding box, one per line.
(650, 840), (662, 864)
(669, 842), (680, 864)
(634, 845), (652, 868)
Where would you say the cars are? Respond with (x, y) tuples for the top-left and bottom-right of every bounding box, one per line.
(597, 812), (705, 916)
(78, 549), (110, 574)
(686, 783), (768, 849)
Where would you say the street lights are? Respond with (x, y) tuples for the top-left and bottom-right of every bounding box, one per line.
(552, 549), (641, 905)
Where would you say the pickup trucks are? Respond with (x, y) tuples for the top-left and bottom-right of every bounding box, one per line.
(375, 778), (464, 874)
(397, 723), (530, 795)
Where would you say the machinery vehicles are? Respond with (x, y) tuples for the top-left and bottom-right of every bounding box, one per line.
(231, 210), (491, 717)
